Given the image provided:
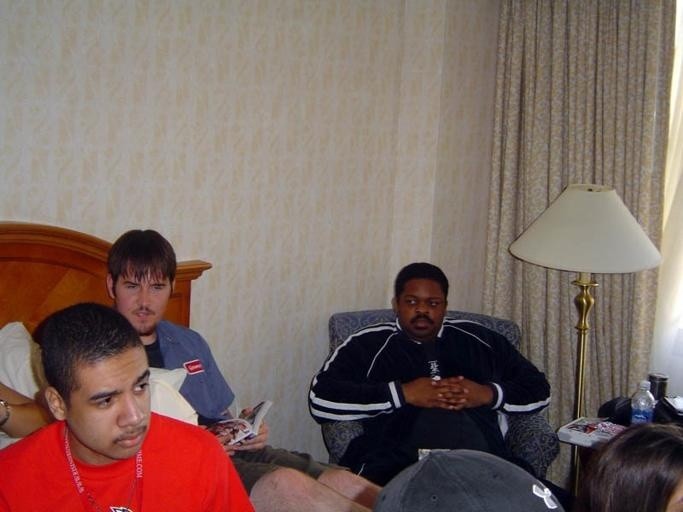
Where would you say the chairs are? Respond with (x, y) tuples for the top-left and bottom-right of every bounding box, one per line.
(321, 308), (561, 482)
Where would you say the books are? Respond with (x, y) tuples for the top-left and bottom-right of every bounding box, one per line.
(205, 400), (273, 447)
(558, 417), (611, 440)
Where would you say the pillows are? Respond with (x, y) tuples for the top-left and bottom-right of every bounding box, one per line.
(0, 320), (201, 451)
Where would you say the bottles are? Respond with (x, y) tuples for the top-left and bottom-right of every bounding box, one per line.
(630, 380), (656, 423)
(648, 372), (668, 400)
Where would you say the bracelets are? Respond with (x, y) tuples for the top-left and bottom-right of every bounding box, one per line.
(0, 398), (10, 428)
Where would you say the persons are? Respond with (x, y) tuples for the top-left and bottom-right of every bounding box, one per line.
(0, 383), (37, 407)
(308, 262), (578, 512)
(577, 422), (681, 512)
(0, 303), (256, 512)
(0, 230), (384, 512)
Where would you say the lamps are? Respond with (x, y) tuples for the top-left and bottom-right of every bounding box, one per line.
(508, 185), (661, 497)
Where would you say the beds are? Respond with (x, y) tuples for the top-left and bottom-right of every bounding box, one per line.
(0, 222), (212, 446)
